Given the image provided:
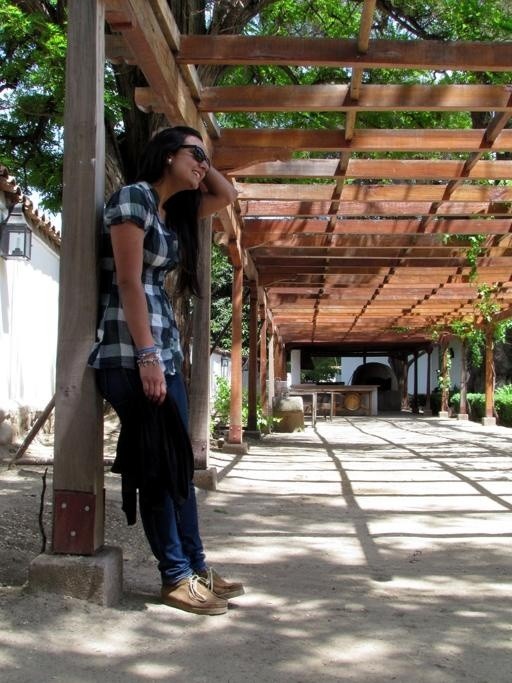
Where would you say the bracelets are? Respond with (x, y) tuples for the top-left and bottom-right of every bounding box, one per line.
(137, 346), (159, 367)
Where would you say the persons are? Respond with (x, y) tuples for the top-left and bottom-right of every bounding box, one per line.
(86, 126), (245, 615)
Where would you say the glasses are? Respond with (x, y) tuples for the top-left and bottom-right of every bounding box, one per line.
(179, 145), (210, 168)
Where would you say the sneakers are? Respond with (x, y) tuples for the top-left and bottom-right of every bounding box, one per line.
(160, 567), (245, 615)
